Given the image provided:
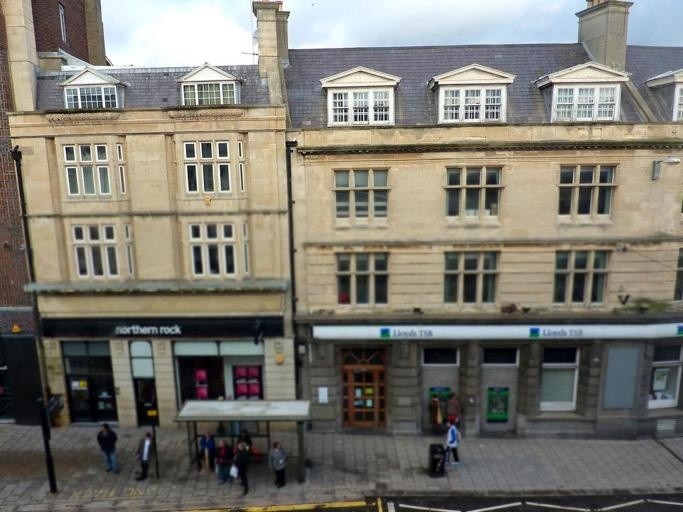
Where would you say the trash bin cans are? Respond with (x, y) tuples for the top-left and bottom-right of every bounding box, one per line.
(429, 443), (446, 477)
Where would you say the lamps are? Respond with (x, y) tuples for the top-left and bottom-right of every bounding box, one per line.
(652, 158), (680, 179)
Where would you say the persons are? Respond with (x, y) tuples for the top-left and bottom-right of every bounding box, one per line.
(198, 427), (289, 496)
(444, 419), (461, 466)
(443, 392), (461, 435)
(133, 431), (156, 482)
(96, 422), (120, 474)
(428, 395), (443, 437)
